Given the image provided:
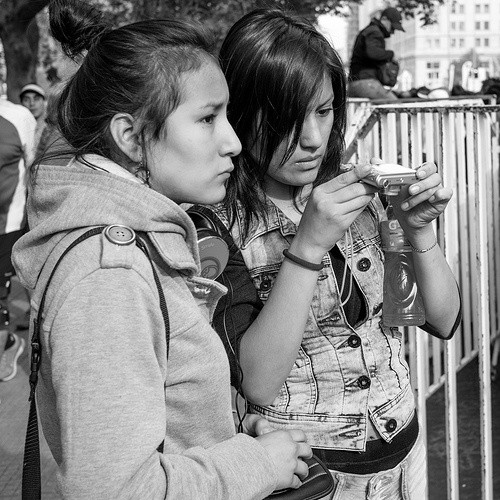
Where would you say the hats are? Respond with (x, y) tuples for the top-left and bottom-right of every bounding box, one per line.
(18, 83), (46, 100)
(382, 8), (405, 32)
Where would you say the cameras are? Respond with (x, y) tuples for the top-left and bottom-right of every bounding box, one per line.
(356, 162), (423, 190)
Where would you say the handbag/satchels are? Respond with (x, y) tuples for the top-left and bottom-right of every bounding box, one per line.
(377, 60), (399, 87)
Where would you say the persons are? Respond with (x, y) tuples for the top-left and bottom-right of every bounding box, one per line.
(11, 15), (313, 499)
(344, 6), (402, 103)
(18, 80), (54, 154)
(178, 5), (467, 500)
(384, 63), (500, 182)
(0, 65), (42, 337)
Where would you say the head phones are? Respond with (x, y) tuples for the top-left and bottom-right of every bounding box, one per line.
(67, 153), (229, 284)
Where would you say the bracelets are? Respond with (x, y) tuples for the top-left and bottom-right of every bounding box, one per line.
(406, 232), (438, 252)
(282, 246), (327, 275)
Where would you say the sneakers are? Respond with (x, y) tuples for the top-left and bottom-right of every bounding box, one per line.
(0, 333), (26, 382)
